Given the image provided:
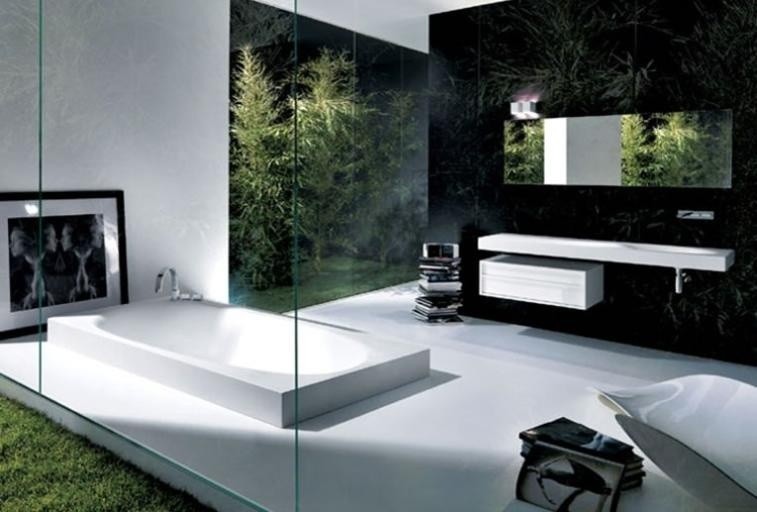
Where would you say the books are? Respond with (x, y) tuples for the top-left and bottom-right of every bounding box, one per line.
(410, 257), (464, 324)
(515, 416), (645, 512)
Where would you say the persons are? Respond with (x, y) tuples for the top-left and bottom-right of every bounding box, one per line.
(8, 217), (107, 312)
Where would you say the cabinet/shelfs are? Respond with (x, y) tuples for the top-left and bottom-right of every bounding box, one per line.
(479, 255), (603, 310)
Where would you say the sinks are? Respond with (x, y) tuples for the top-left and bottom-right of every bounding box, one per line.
(630, 244), (710, 254)
(47, 292), (430, 430)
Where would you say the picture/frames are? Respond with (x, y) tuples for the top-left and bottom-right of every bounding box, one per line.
(0, 191), (39, 341)
(42, 190), (128, 344)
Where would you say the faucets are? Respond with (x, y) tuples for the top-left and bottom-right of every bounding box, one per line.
(153, 262), (205, 303)
